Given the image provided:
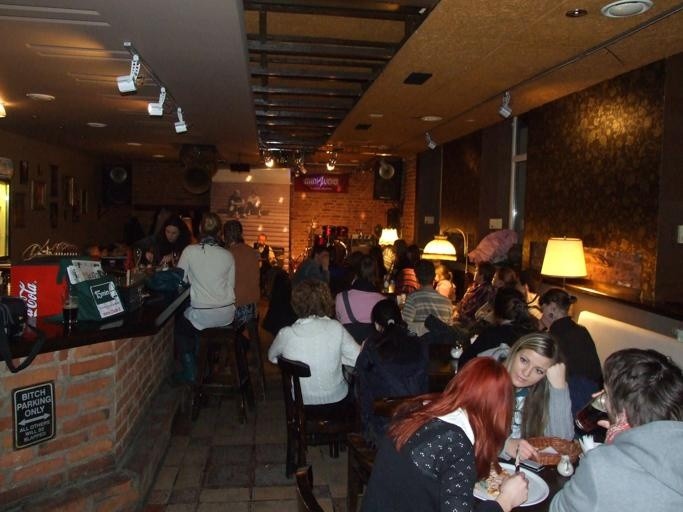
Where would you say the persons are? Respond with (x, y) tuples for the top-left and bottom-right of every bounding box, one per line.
(549, 347), (683, 512)
(267, 239), (537, 372)
(266, 278), (361, 421)
(362, 356), (529, 512)
(474, 332), (575, 464)
(246, 190), (262, 217)
(538, 288), (603, 419)
(226, 189), (248, 219)
(351, 298), (431, 433)
(131, 210), (277, 388)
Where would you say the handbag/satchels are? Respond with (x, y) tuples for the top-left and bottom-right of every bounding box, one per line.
(0, 296), (46, 373)
(144, 267), (185, 293)
(55, 256), (125, 325)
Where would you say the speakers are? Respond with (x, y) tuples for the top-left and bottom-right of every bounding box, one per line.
(102, 161), (132, 207)
(373, 156), (406, 202)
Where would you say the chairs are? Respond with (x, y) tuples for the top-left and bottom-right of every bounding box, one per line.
(294, 464), (326, 512)
(278, 357), (352, 477)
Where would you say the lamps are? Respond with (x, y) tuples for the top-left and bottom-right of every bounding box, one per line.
(147, 87), (166, 116)
(421, 228), (470, 274)
(379, 228), (399, 246)
(424, 133), (437, 150)
(117, 55), (141, 94)
(378, 159), (394, 179)
(327, 159), (337, 171)
(173, 106), (188, 133)
(265, 156), (273, 169)
(541, 236), (589, 291)
(294, 158), (309, 175)
(498, 92), (513, 119)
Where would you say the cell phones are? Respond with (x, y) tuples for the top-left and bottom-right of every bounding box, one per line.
(519, 459), (545, 473)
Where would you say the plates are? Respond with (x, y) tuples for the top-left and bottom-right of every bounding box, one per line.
(473, 461), (550, 507)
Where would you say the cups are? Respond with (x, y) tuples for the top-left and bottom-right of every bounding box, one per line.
(576, 390), (607, 432)
(62, 295), (78, 328)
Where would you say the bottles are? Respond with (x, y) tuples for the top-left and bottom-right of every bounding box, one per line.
(557, 456), (574, 477)
(451, 341), (463, 359)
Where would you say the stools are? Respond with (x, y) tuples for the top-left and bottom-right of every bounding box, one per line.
(181, 321), (254, 422)
(347, 436), (381, 512)
(242, 317), (267, 403)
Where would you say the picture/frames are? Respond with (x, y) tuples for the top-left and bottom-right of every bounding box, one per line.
(13, 192), (28, 225)
(33, 181), (49, 209)
(50, 202), (60, 227)
